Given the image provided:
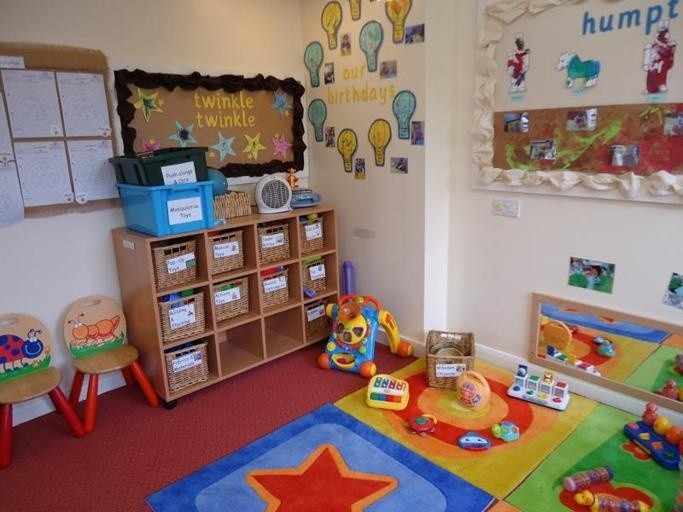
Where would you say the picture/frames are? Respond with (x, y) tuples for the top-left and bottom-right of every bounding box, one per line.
(529, 291), (683, 415)
(113, 69), (306, 186)
(471, 2), (683, 206)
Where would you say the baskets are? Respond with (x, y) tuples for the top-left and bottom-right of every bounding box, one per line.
(158, 291), (206, 343)
(213, 189), (252, 219)
(300, 216), (325, 255)
(165, 340), (209, 394)
(301, 258), (327, 292)
(212, 275), (249, 322)
(257, 224), (290, 264)
(425, 330), (476, 389)
(152, 239), (198, 290)
(303, 299), (329, 337)
(209, 230), (244, 275)
(260, 266), (290, 309)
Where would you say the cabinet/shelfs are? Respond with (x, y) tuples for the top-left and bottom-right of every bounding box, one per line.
(112, 224), (265, 408)
(255, 205), (339, 366)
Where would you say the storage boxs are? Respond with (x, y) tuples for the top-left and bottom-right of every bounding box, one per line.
(109, 146), (216, 185)
(116, 180), (215, 236)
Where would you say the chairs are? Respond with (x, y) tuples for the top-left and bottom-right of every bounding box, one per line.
(0, 313), (86, 471)
(63, 297), (158, 431)
